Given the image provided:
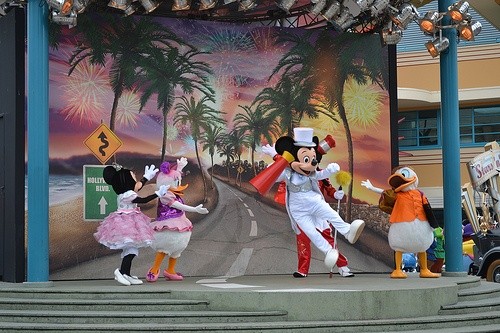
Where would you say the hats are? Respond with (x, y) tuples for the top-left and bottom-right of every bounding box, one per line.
(462, 223), (474, 237)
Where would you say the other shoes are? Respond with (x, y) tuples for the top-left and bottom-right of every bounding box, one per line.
(337, 265), (354, 277)
(292, 271), (307, 278)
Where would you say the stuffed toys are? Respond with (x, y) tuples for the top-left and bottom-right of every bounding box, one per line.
(93, 166), (170, 287)
(262, 128), (365, 278)
(361, 167), (443, 278)
(146, 157), (209, 281)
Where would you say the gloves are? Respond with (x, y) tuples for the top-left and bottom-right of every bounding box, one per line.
(334, 189), (344, 200)
(262, 143), (278, 159)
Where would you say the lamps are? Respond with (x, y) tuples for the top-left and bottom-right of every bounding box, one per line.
(0, 0), (89, 29)
(355, 0), (374, 13)
(339, 13), (359, 32)
(459, 20), (482, 41)
(369, 0), (399, 17)
(333, 6), (349, 26)
(198, 0), (215, 10)
(322, 0), (340, 21)
(172, 0), (191, 11)
(380, 23), (403, 48)
(139, 0), (160, 13)
(124, 3), (135, 16)
(309, 0), (327, 16)
(424, 29), (451, 59)
(107, 0), (127, 10)
(389, 2), (419, 27)
(448, 0), (470, 23)
(418, 10), (444, 33)
(238, 0), (258, 11)
(273, 0), (296, 12)
